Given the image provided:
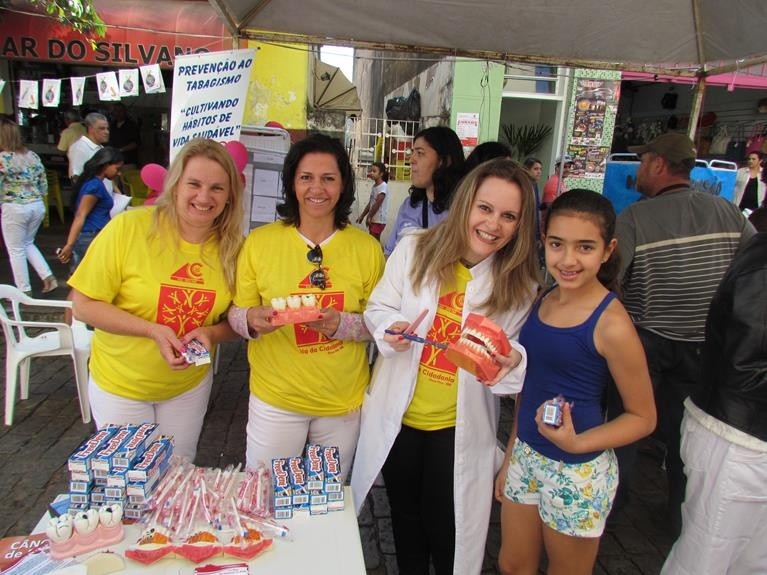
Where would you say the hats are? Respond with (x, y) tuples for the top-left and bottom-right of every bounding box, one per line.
(627, 132), (699, 164)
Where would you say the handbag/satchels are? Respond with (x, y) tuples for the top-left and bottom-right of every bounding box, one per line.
(725, 125), (746, 163)
(708, 126), (730, 154)
(744, 122), (763, 160)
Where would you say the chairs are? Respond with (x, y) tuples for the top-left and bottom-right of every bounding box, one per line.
(39, 170), (68, 232)
(0, 283), (96, 427)
(120, 164), (158, 209)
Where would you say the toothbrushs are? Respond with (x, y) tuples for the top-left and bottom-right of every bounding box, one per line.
(384, 328), (449, 350)
(181, 351), (194, 364)
(135, 457), (289, 548)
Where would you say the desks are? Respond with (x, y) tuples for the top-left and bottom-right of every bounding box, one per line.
(7, 486), (368, 574)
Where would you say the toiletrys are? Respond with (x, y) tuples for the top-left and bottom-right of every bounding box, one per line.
(268, 441), (346, 520)
(541, 401), (564, 427)
(186, 339), (212, 367)
(66, 421), (175, 519)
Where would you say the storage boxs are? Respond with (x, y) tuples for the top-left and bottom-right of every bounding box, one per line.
(177, 335), (212, 367)
(542, 401), (563, 430)
(48, 419), (181, 524)
(272, 441), (345, 520)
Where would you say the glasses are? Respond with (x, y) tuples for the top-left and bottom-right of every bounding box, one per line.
(306, 244), (326, 290)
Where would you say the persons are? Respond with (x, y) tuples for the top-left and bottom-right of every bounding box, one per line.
(355, 163), (390, 242)
(349, 157), (542, 575)
(736, 151), (767, 219)
(656, 233), (767, 575)
(390, 128), (575, 261)
(59, 109), (124, 274)
(227, 133), (386, 486)
(0, 117), (57, 295)
(617, 132), (756, 537)
(493, 189), (657, 575)
(68, 138), (247, 461)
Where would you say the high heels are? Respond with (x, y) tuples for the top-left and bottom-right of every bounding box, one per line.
(41, 275), (58, 296)
